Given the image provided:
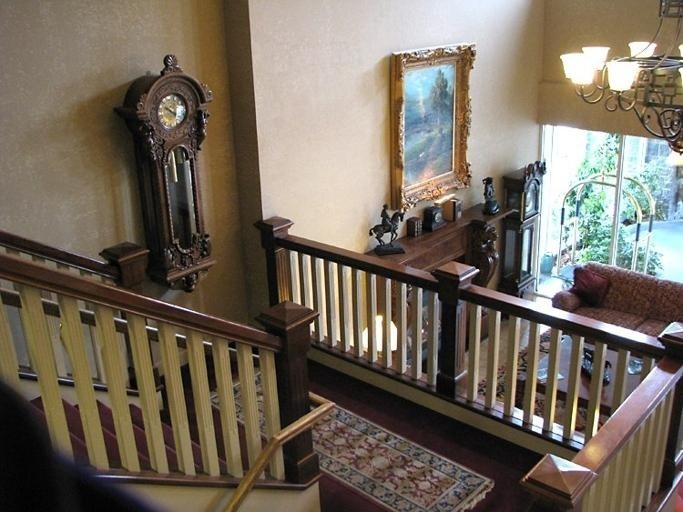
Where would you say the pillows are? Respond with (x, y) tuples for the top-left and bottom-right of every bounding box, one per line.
(572, 267), (608, 307)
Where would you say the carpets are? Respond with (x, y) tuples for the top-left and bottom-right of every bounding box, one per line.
(210, 366), (495, 512)
(477, 329), (610, 437)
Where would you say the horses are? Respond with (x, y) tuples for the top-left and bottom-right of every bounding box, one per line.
(369, 212), (405, 247)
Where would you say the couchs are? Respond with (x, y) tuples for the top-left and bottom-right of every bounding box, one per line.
(552, 261), (683, 339)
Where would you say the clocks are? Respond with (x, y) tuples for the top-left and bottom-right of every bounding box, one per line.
(112, 52), (218, 293)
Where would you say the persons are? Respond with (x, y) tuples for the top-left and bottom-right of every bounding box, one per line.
(482, 176), (495, 203)
(380, 204), (393, 234)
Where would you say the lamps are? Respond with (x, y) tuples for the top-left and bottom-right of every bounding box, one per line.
(560, 0), (683, 156)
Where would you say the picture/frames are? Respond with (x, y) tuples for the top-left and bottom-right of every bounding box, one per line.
(391, 41), (477, 212)
